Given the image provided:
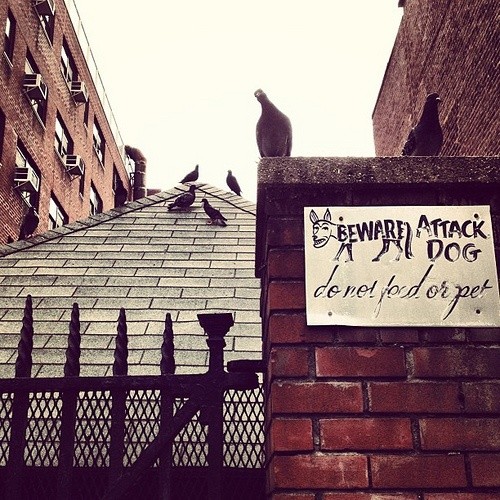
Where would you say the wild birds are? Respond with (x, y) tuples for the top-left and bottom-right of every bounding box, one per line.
(166, 185), (198, 212)
(254, 89), (293, 158)
(200, 199), (227, 226)
(226, 169), (243, 198)
(178, 164), (199, 185)
(402, 93), (443, 157)
